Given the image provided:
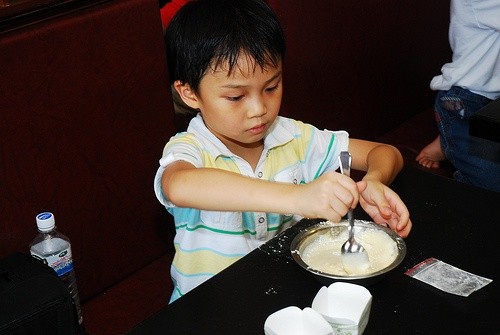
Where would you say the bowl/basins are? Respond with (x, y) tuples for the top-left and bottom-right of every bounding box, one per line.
(290, 218), (407, 279)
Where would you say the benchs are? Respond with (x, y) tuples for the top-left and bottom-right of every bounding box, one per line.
(268, 2), (452, 165)
(1, 0), (178, 335)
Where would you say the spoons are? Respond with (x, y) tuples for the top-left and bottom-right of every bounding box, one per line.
(337, 150), (369, 262)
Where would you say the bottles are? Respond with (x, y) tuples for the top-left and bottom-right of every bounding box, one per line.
(29, 213), (84, 324)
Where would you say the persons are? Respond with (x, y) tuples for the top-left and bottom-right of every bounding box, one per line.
(153, 0), (412, 309)
(414, 0), (500, 194)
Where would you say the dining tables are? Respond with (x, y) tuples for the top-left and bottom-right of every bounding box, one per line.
(128, 171), (499, 335)
